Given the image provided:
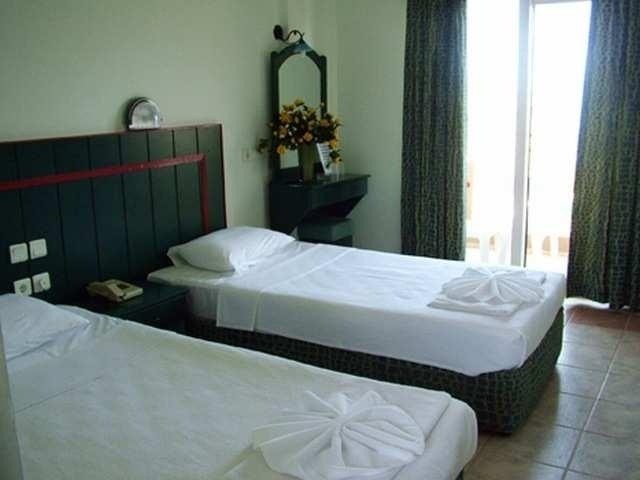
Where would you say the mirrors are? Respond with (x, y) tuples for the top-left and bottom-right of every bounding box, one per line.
(269, 44), (327, 185)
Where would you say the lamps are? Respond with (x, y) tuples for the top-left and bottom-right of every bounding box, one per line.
(271, 23), (313, 58)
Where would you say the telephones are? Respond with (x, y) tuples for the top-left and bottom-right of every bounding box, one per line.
(85, 279), (144, 303)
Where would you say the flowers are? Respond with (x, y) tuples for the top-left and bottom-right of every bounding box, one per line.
(259, 102), (345, 165)
